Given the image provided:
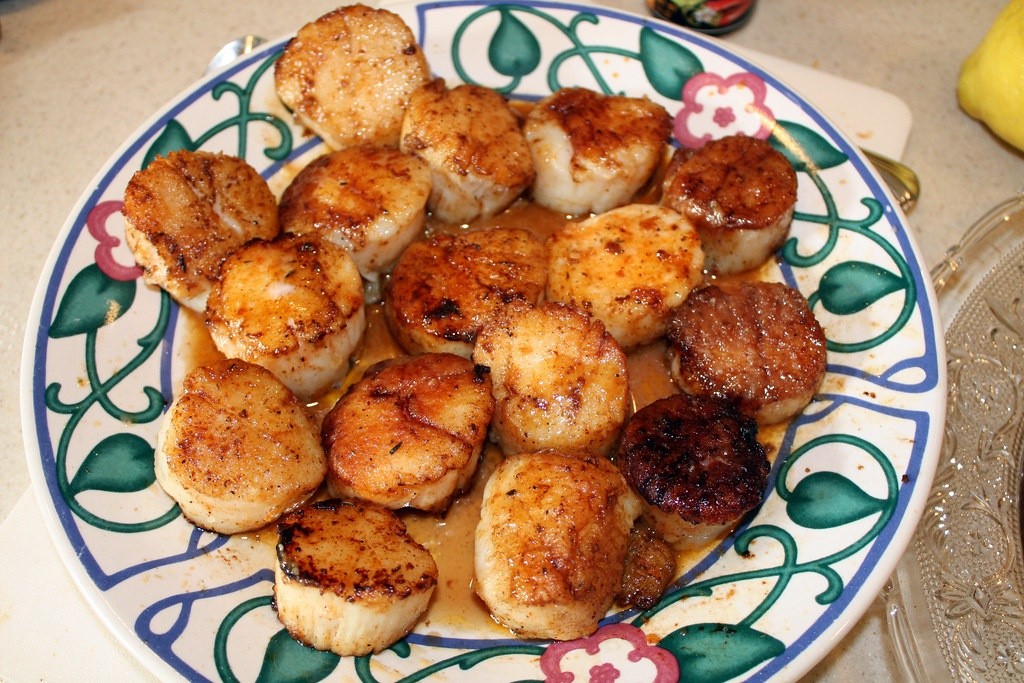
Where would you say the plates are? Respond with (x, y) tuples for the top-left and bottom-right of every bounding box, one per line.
(20, 0), (949, 683)
(884, 200), (1024, 682)
